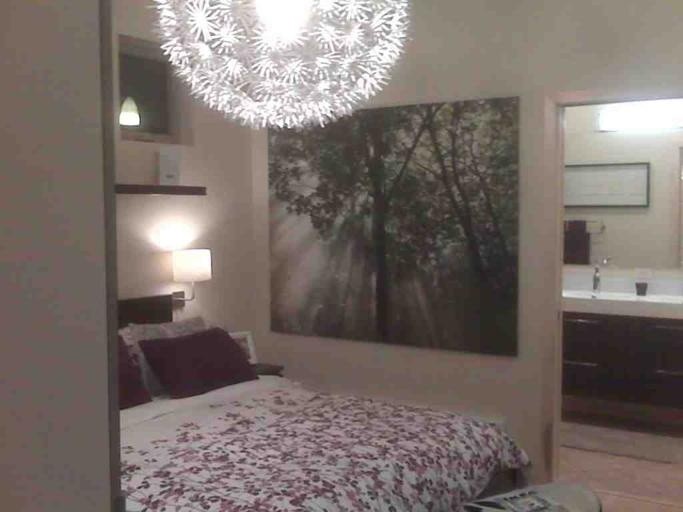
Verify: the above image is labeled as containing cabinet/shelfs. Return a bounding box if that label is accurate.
[563,311,682,409]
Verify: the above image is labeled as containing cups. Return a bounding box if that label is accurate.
[635,282,648,296]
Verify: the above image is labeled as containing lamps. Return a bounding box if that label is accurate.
[170,248,213,309]
[150,0,409,135]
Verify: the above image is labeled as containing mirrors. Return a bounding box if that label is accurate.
[564,130,683,270]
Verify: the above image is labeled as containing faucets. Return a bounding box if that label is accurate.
[593,264,601,298]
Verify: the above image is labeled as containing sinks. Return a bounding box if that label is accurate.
[563,290,637,301]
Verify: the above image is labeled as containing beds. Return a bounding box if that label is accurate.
[119,294,527,512]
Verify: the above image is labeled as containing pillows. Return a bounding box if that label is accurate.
[119,315,258,410]
